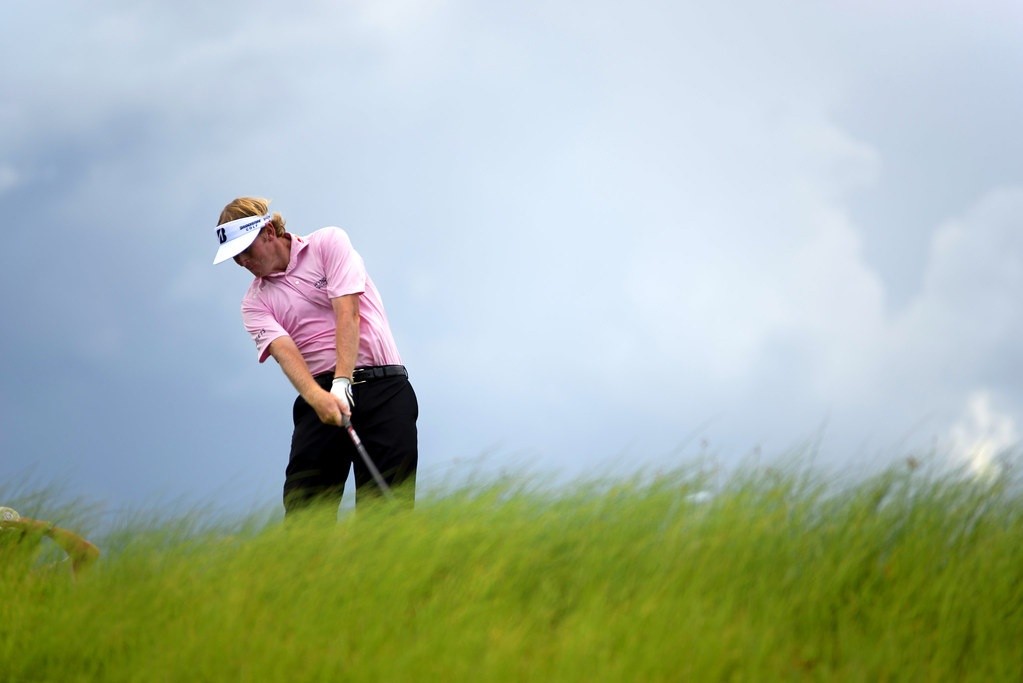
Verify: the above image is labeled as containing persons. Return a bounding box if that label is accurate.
[212,197,419,531]
[0,506,101,589]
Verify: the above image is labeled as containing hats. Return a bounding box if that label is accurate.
[213,212,272,265]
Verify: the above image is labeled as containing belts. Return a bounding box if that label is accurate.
[351,365,407,384]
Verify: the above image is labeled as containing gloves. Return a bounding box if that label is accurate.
[330,379,354,414]
